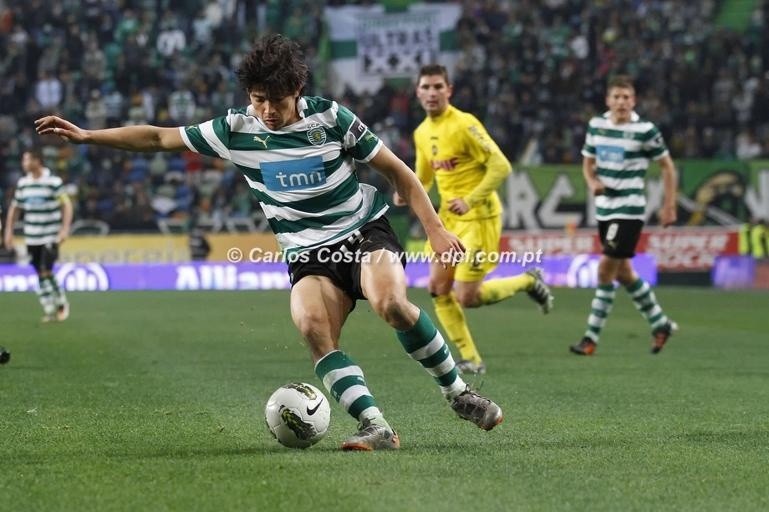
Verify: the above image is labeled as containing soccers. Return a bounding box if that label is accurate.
[263,382,331,448]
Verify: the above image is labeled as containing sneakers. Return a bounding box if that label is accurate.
[456,361,489,376]
[652,319,678,354]
[526,267,554,315]
[569,337,596,356]
[450,390,504,432]
[341,417,400,451]
[39,295,70,322]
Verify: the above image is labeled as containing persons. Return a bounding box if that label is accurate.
[0,0,768,264]
[33,33,504,449]
[567,74,678,356]
[393,63,554,375]
[2,147,73,322]
